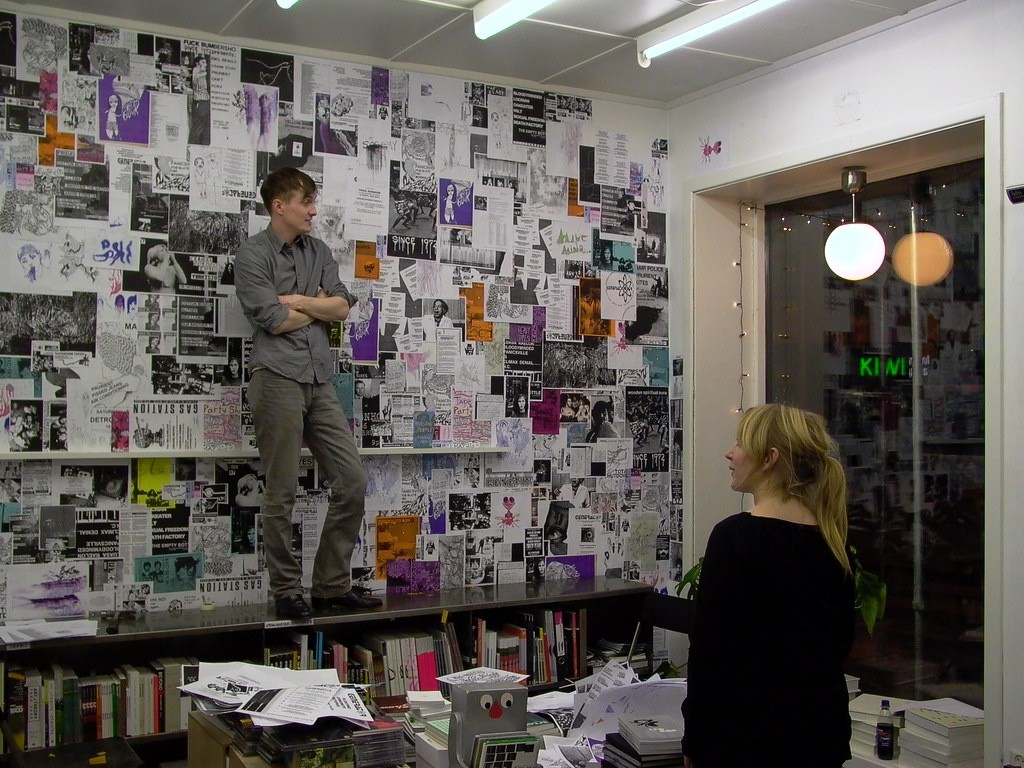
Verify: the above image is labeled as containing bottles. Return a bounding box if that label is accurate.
[875,700,894,761]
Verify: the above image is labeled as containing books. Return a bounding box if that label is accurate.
[0,602,685,768]
[844,674,985,768]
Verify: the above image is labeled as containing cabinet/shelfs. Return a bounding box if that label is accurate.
[0,577,654,754]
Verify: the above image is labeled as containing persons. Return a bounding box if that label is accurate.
[511,393,528,419]
[534,393,671,580]
[423,300,453,340]
[143,244,187,295]
[233,167,383,620]
[681,403,855,768]
[220,357,242,386]
[238,525,256,554]
[221,261,236,285]
[391,177,519,245]
[598,246,616,271]
[235,473,265,507]
[355,376,386,415]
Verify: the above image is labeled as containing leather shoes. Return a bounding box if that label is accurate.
[311,591,383,612]
[275,594,314,620]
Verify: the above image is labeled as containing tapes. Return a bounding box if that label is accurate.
[200,602,214,611]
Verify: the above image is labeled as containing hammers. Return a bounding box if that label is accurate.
[100,608,136,634]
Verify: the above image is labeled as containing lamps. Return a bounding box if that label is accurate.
[824,166,885,281]
[473,0,557,40]
[636,0,785,68]
[891,172,952,288]
